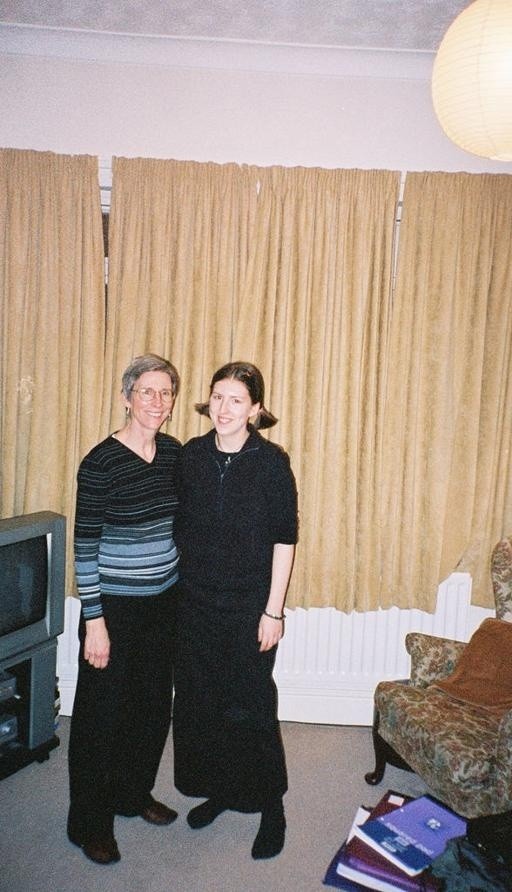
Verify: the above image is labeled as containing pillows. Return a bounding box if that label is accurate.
[425,617,512,714]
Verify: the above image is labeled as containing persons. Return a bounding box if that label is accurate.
[72,353,177,868]
[173,364,299,860]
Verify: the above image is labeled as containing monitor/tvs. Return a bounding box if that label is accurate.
[0,509,69,662]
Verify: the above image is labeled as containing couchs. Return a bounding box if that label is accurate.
[364,533,512,852]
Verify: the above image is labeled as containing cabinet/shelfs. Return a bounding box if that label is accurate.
[0,637,60,781]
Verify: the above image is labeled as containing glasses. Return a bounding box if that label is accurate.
[127,386,177,402]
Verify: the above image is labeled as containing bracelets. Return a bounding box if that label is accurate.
[262,610,286,620]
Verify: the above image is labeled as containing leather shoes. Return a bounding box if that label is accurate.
[66,829,121,864]
[116,795,178,826]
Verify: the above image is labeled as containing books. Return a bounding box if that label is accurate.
[321,789,468,891]
